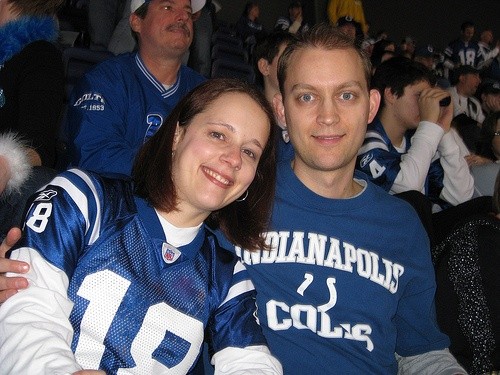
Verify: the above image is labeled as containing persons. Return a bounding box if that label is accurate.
[1,0,500,233]
[0,20,467,375]
[0,76,279,375]
[66,0,208,178]
